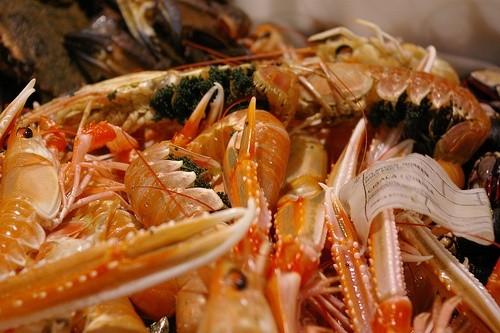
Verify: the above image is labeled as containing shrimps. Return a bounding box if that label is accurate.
[0,18,500,333]
